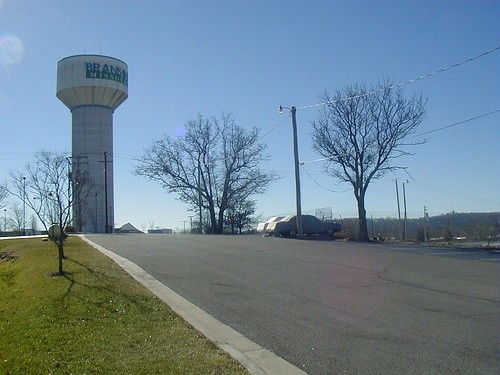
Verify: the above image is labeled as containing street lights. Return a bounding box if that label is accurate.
[97,151,113,233]
[4,209,6,231]
[278,105,303,239]
[22,176,26,236]
[402,179,408,239]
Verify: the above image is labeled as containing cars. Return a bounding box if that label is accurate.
[256,216,286,236]
[266,214,342,238]
